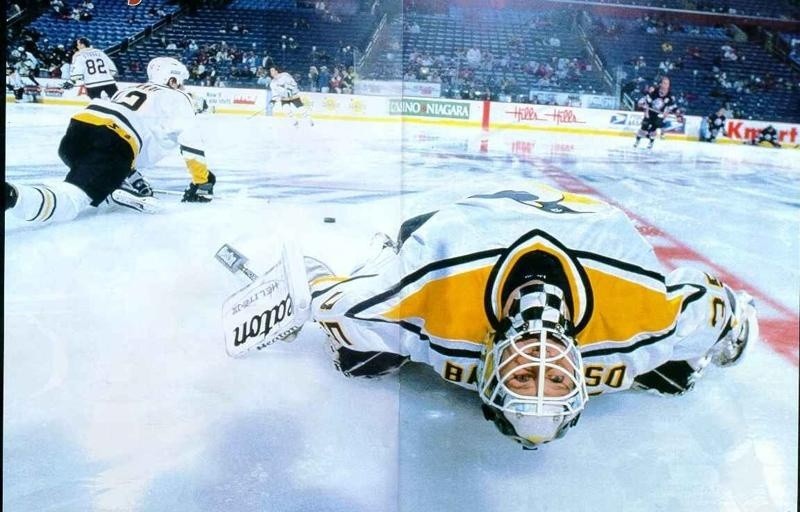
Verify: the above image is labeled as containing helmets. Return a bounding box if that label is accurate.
[476,324,591,453]
[146,57,190,86]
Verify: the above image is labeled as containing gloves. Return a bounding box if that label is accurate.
[181,170,215,202]
[62,78,76,90]
[129,171,154,196]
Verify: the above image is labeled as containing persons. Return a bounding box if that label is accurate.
[4,56,217,224]
[302,177,759,451]
[4,0,799,150]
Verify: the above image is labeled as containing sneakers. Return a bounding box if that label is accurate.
[112,187,162,213]
[634,141,654,148]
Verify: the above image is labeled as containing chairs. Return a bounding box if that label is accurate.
[27,1,800,130]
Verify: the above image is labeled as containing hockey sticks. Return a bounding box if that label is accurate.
[153,186,249,201]
[47,82,84,89]
[244,103,271,120]
